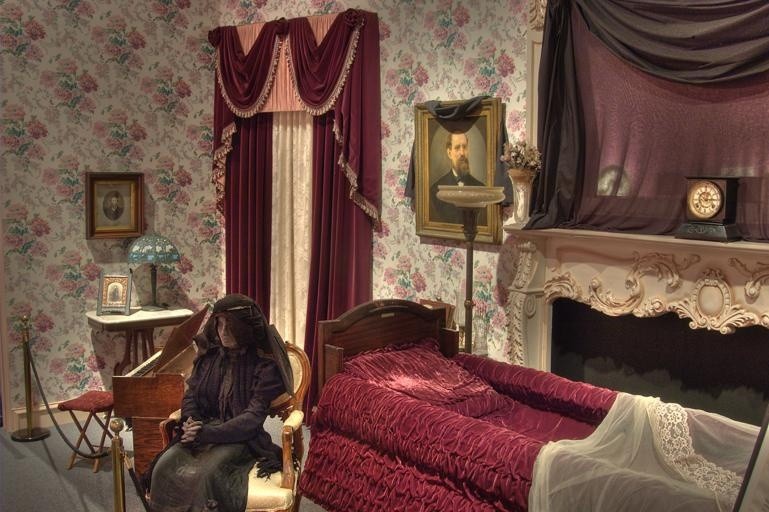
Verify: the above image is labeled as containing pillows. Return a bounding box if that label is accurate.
[343,337,507,418]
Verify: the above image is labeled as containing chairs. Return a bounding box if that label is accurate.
[159,341,311,512]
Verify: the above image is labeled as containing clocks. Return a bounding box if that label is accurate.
[673,176,741,242]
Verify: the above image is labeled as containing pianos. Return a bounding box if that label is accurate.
[111,304,210,483]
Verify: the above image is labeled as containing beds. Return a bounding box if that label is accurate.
[297,298,768,511]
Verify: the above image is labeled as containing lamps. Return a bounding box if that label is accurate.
[128,233,181,311]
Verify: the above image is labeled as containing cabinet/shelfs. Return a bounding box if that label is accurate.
[414,98,505,248]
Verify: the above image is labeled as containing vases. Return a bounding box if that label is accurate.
[508,169,539,225]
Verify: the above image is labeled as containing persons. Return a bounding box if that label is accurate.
[104,191,123,220]
[149,293,293,512]
[430,129,487,226]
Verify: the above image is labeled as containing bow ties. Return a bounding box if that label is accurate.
[455,174,467,183]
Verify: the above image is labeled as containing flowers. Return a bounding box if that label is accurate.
[499,139,542,170]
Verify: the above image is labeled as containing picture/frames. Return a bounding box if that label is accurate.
[83,171,145,241]
[95,271,133,315]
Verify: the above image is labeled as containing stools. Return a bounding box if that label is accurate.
[57,390,122,473]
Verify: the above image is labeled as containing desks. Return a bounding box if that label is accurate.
[85,306,193,376]
